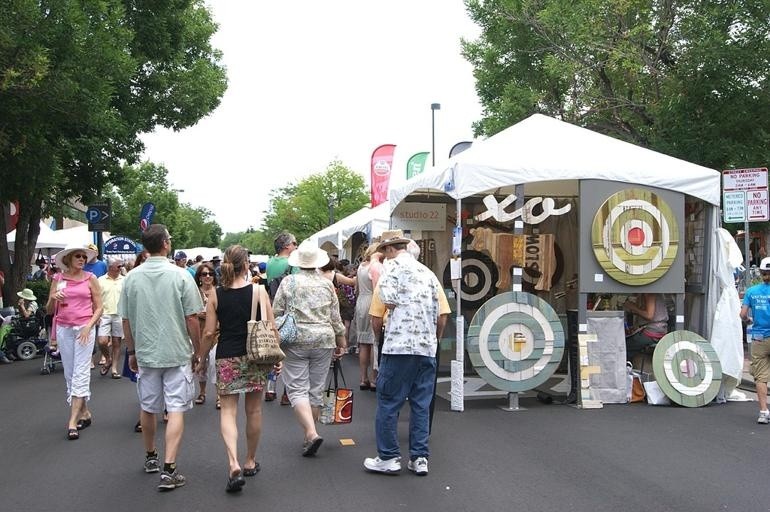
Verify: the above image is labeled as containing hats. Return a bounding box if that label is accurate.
[377,229,410,253]
[17,288,37,300]
[759,257,770,269]
[288,240,329,268]
[210,256,222,261]
[173,251,186,260]
[55,242,97,270]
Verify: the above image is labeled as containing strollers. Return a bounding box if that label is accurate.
[39,314,61,374]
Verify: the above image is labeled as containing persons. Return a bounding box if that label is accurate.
[46,241,103,440]
[195,245,280,491]
[363,229,439,473]
[750,238,762,263]
[119,225,204,489]
[0,249,452,436]
[738,255,770,424]
[623,293,670,368]
[266,233,300,302]
[271,242,347,455]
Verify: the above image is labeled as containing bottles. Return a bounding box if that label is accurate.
[266,370,277,395]
[745,321,754,344]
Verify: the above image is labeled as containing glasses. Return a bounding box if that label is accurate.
[200,271,214,277]
[74,254,88,259]
[117,264,125,268]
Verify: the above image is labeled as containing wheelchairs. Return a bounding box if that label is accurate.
[1,307,46,361]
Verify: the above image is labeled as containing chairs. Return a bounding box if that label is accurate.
[639,314,676,375]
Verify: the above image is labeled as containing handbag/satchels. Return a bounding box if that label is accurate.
[273,314,297,343]
[270,270,292,299]
[627,371,671,404]
[247,320,286,365]
[36,257,46,265]
[318,388,353,424]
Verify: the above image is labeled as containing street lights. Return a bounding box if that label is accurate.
[174,187,186,197]
[325,193,336,225]
[429,102,442,168]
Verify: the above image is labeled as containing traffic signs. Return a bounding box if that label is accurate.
[720,167,769,191]
[746,189,770,222]
[85,196,112,232]
[722,190,747,224]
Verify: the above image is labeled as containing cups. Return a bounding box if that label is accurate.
[60,293,69,308]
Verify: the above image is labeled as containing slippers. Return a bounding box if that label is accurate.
[303,435,323,456]
[99,359,122,379]
[68,418,91,438]
[226,475,245,492]
[244,462,260,475]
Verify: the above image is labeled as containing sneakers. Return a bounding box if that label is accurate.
[216,397,221,409]
[360,377,377,391]
[158,472,185,488]
[281,394,290,404]
[757,409,770,423]
[265,391,277,400]
[363,455,401,473]
[407,457,428,473]
[195,393,205,403]
[144,455,162,472]
